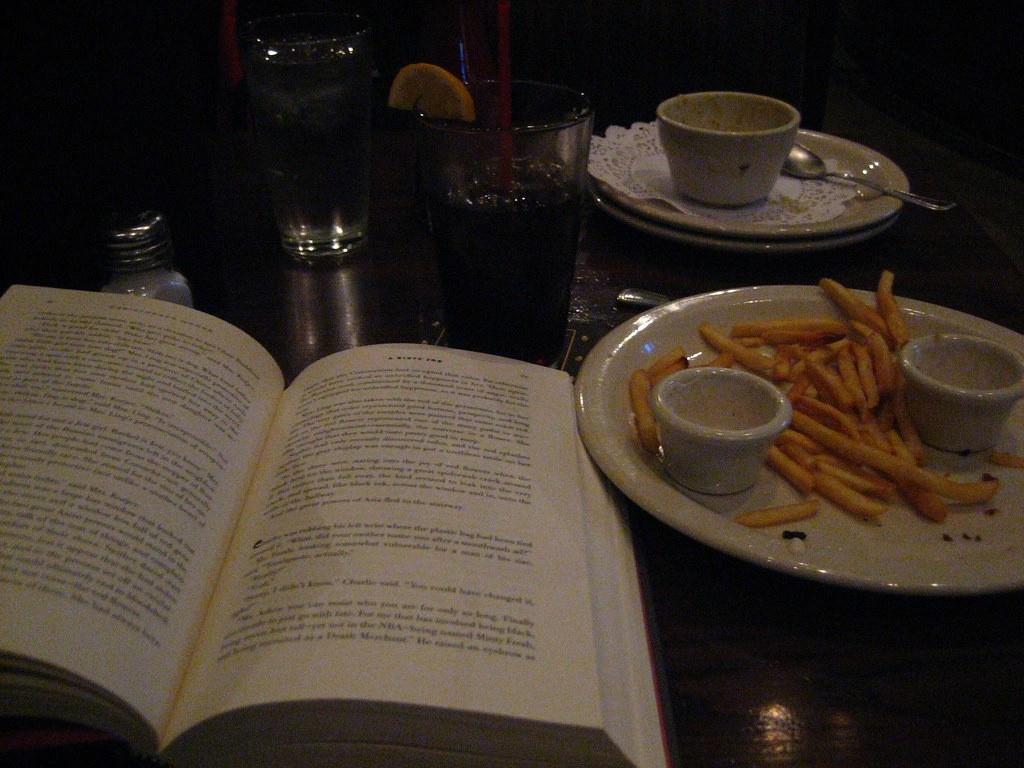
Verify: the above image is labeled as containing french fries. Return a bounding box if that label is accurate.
[628,268,1024,529]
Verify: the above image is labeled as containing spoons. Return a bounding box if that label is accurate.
[781,143,956,210]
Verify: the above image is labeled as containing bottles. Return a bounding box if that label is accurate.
[86,209,193,310]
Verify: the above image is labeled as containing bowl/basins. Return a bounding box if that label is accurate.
[648,367,791,495]
[897,332,1024,453]
[656,92,799,206]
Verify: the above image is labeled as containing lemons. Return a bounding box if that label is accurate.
[388,62,476,121]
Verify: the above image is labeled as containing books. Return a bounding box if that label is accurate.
[0,283,671,768]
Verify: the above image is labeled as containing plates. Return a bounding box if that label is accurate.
[588,128,911,249]
[572,284,1024,596]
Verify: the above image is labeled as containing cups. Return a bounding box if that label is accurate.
[417,80,596,364]
[241,11,375,257]
